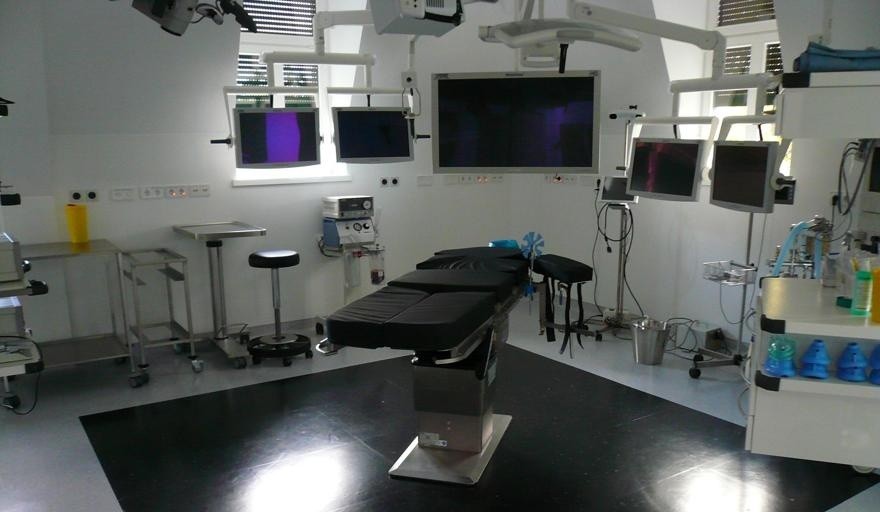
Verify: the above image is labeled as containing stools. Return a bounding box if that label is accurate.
[531,255,602,354]
[246,250,313,367]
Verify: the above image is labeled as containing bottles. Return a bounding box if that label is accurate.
[868,263,880,324]
[849,260,871,316]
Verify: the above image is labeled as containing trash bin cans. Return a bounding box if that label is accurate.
[632,319,668,365]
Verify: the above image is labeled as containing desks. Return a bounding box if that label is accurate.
[172,221,266,368]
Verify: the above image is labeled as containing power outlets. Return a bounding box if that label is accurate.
[544,175,577,185]
[593,175,605,188]
[140,185,210,199]
[460,173,504,185]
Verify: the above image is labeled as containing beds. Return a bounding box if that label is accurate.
[316,245,533,484]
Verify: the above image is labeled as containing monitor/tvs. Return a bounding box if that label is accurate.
[626,137,704,202]
[429,70,603,176]
[597,175,639,206]
[332,106,414,165]
[710,140,778,214]
[232,107,321,168]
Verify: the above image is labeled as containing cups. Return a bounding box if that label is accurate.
[64,202,90,245]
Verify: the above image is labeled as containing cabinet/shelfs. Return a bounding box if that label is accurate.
[0,97,48,410]
[744,68,879,474]
